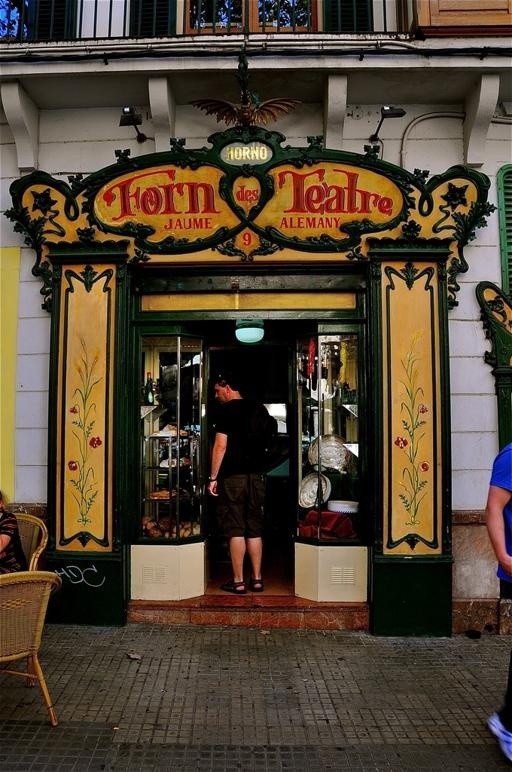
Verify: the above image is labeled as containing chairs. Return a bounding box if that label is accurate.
[0,571,65,727]
[13,511,50,571]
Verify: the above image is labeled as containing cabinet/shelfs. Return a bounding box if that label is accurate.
[286,321,368,604]
[124,324,210,602]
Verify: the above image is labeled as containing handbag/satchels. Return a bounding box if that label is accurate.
[239,406,279,474]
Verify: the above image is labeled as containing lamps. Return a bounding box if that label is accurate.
[368,104,407,148]
[116,103,149,145]
[235,318,266,344]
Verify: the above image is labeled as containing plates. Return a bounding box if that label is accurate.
[307,435,350,473]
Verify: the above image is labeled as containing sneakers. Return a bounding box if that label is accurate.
[486,711,512,762]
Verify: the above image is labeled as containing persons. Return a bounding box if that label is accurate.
[0,489,29,575]
[485,442,512,762]
[207,375,273,594]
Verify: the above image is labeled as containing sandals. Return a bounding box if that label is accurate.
[221,577,249,593]
[247,577,264,592]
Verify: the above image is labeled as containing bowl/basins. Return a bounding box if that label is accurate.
[158,473,168,483]
[300,473,331,508]
[328,501,359,514]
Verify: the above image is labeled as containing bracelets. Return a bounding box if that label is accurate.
[210,479,217,482]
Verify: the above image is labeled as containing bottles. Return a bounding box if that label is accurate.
[144,372,157,406]
